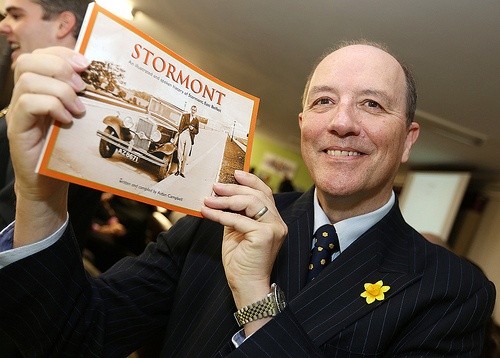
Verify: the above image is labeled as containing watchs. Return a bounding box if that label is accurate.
[234,282,287,327]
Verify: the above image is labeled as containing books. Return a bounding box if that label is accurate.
[32,1,261,223]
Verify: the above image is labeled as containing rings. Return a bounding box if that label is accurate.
[254,206,268,220]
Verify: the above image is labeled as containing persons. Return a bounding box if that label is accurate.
[174,105,199,179]
[0,0,98,232]
[0,44,497,358]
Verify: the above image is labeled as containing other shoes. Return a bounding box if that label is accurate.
[175,171,179,176]
[180,173,185,178]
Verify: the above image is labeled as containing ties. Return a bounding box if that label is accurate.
[306,224,341,284]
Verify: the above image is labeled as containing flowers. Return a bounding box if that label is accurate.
[360,280,391,304]
